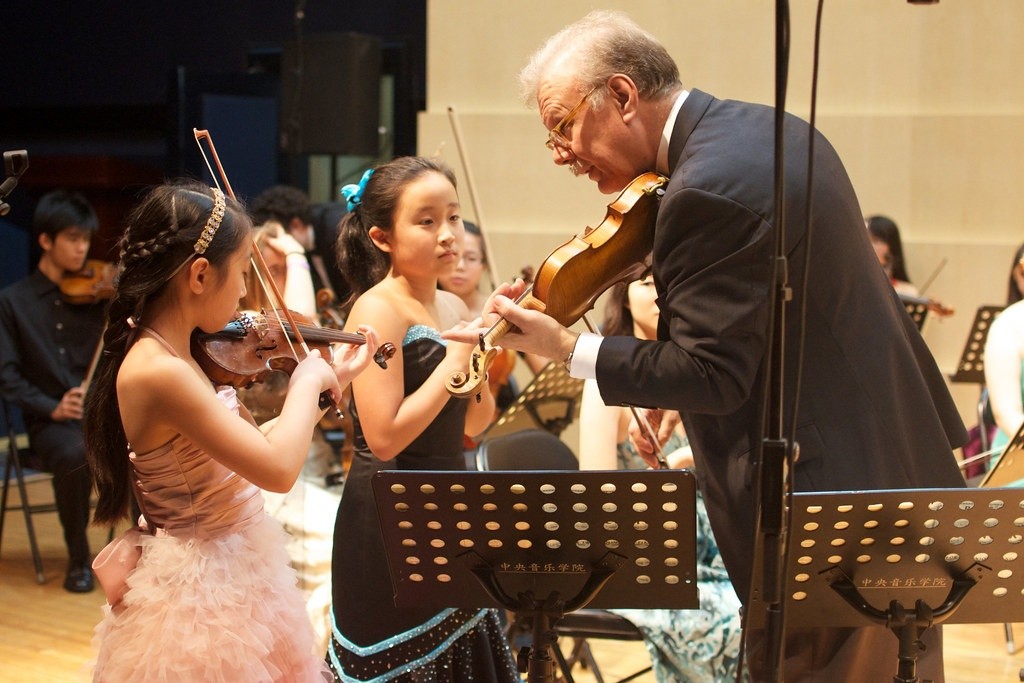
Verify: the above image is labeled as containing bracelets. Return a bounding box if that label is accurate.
[285,252,305,256]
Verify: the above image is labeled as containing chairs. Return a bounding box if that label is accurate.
[476,429,646,683]
[0,392,118,584]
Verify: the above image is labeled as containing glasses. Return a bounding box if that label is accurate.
[456,252,485,267]
[546,85,606,152]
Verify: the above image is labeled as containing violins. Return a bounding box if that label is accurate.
[926,299,954,317]
[462,348,516,451]
[190,307,397,389]
[59,259,118,304]
[445,171,671,404]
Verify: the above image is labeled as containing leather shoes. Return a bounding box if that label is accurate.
[64,555,94,592]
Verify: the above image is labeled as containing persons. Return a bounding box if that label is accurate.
[578,275,749,683]
[239,221,316,321]
[0,193,140,593]
[440,15,968,683]
[982,239,1024,487]
[80,182,379,683]
[250,186,358,312]
[436,221,518,409]
[324,158,519,683]
[863,215,920,309]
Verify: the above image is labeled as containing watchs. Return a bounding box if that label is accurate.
[563,333,581,372]
